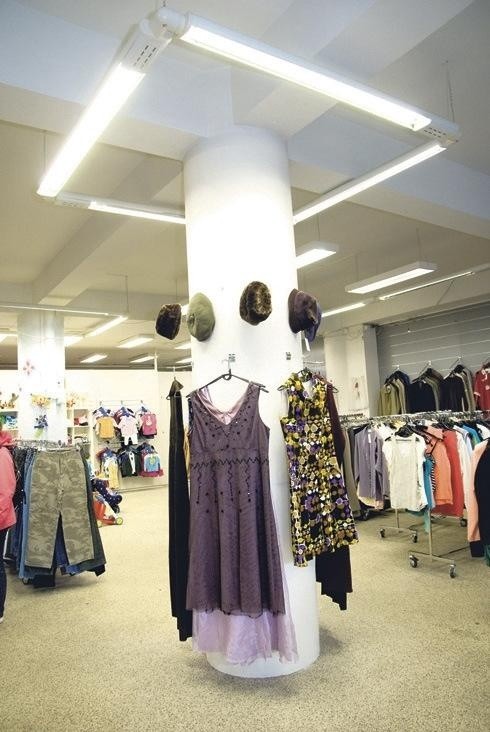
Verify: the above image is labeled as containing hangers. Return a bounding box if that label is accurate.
[419,362,434,376]
[187,356,266,394]
[337,414,364,422]
[482,355,489,367]
[367,414,409,424]
[277,361,338,394]
[399,410,490,423]
[17,439,80,451]
[448,358,461,371]
[387,365,398,383]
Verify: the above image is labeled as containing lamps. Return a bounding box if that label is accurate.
[292,141,448,226]
[35,22,172,201]
[175,276,188,316]
[51,193,187,226]
[173,338,191,351]
[344,263,439,296]
[175,354,190,369]
[152,6,465,147]
[295,244,341,270]
[113,333,156,349]
[71,352,109,363]
[130,351,156,363]
[1,329,84,342]
[85,276,130,338]
[375,270,474,300]
[320,299,369,317]
[0,300,121,319]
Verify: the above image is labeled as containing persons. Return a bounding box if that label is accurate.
[0,422,18,622]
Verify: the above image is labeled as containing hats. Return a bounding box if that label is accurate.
[287,288,318,334]
[239,281,272,326]
[185,293,216,342]
[155,303,182,340]
[304,304,322,343]
